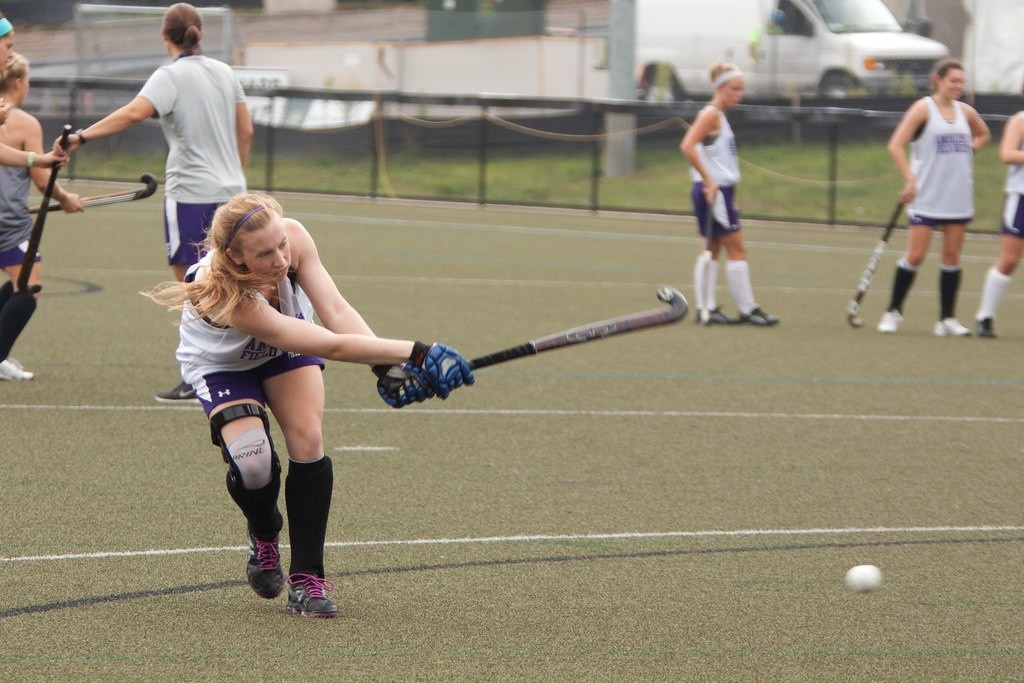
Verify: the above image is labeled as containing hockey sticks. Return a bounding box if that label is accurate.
[845,201,904,327]
[700,204,712,325]
[16,125,73,296]
[27,172,158,215]
[388,286,689,394]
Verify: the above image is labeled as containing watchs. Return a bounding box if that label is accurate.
[75,127,87,144]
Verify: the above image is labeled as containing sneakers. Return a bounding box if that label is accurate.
[246,523,284,599]
[933,317,971,337]
[155,380,200,403]
[287,573,338,619]
[0,357,34,382]
[741,307,779,326]
[976,317,998,338]
[699,308,742,325]
[878,309,904,333]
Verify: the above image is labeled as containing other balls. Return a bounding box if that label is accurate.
[846,564,882,592]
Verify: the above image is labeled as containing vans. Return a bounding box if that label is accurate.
[632,0,954,144]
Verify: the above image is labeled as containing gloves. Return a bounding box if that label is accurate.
[409,341,474,399]
[371,363,435,408]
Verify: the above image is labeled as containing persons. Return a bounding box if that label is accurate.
[138,191,474,619]
[975,89,1024,338]
[680,61,783,327]
[52,1,254,404]
[877,58,992,340]
[0,9,86,385]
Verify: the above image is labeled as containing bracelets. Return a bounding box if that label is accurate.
[27,151,35,168]
